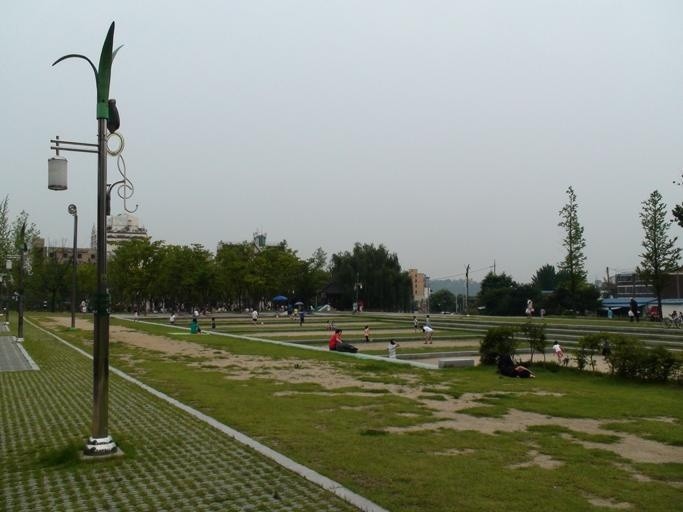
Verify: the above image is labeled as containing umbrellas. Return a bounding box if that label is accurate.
[273,295,288,301]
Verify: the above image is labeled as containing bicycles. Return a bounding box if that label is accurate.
[660,313,682,329]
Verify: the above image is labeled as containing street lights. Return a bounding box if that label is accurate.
[353,271,363,304]
[5,247,25,342]
[65,202,77,328]
[42,20,132,463]
[423,276,431,314]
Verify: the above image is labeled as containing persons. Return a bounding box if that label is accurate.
[628,297,641,322]
[353,300,364,312]
[252,309,258,325]
[413,315,435,344]
[329,329,358,353]
[325,320,336,330]
[170,312,176,325]
[540,308,546,319]
[189,309,202,334]
[388,339,400,359]
[79,298,88,317]
[552,340,564,363]
[608,307,613,318]
[500,366,535,379]
[364,326,369,342]
[287,304,305,328]
[526,297,534,318]
